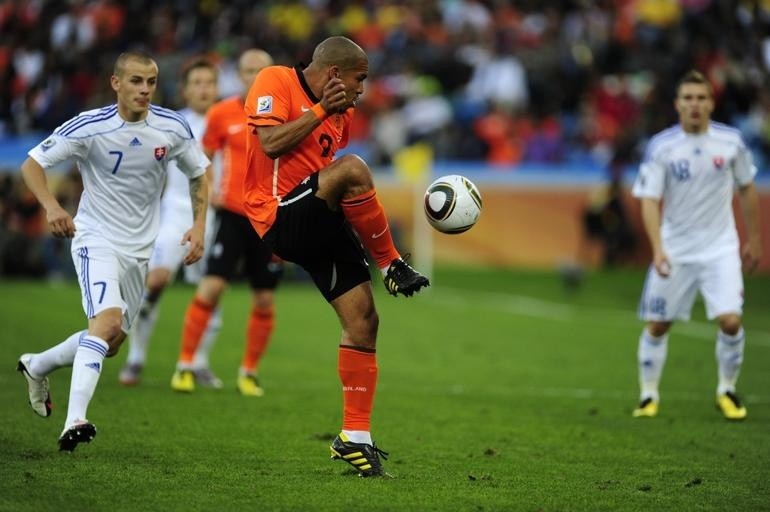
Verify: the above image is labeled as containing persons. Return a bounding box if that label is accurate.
[240,36,431,477]
[628,70,760,422]
[170,49,292,398]
[0,1,769,251]
[117,61,233,392]
[20,49,212,455]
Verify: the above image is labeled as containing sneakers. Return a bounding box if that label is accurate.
[714,391,748,422]
[56,421,97,452]
[14,352,53,418]
[116,361,266,399]
[631,399,660,418]
[379,256,430,298]
[328,430,390,477]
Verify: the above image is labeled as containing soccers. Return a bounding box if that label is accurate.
[424,175,484,235]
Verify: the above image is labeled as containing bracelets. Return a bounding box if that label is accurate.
[310,102,330,122]
